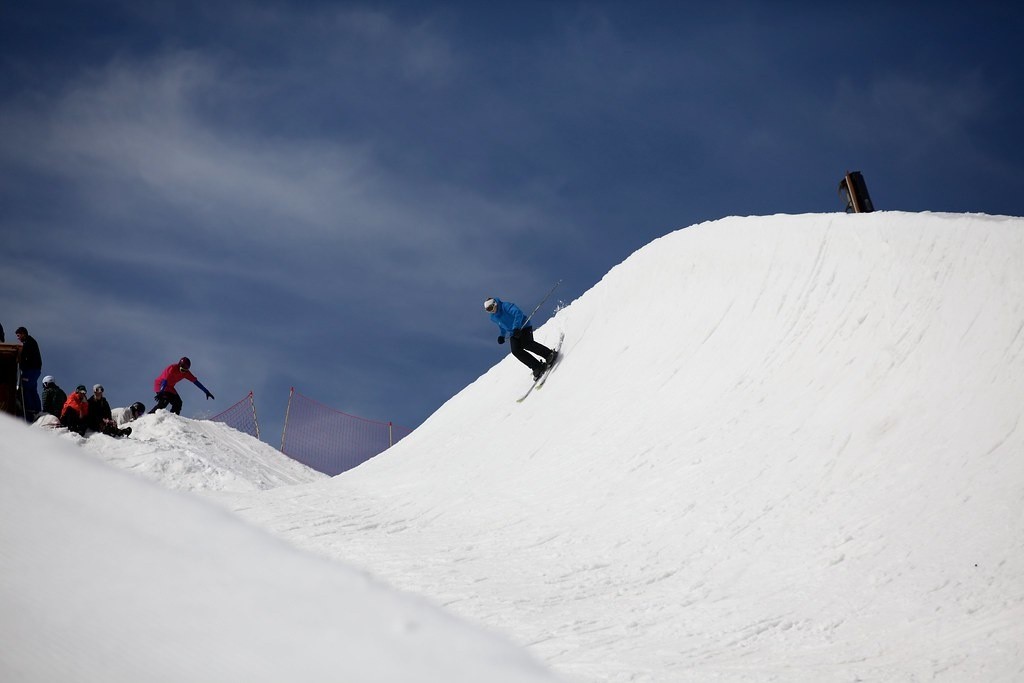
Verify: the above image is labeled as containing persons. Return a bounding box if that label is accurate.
[147,357,214,416]
[111,402,145,426]
[484,298,558,381]
[87,384,132,437]
[41,376,67,418]
[14,327,42,422]
[58,384,88,437]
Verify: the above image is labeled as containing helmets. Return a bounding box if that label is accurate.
[93,384,104,393]
[76,385,87,394]
[177,357,191,368]
[130,402,145,415]
[42,375,56,383]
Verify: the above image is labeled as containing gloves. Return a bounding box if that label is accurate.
[205,392,214,400]
[498,336,505,344]
[153,392,163,402]
[513,328,522,338]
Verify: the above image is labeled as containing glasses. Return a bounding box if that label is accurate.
[483,300,498,311]
[179,366,186,372]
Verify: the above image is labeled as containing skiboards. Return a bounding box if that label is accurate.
[516,332,565,402]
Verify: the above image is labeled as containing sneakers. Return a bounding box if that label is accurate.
[533,359,547,381]
[546,349,559,370]
